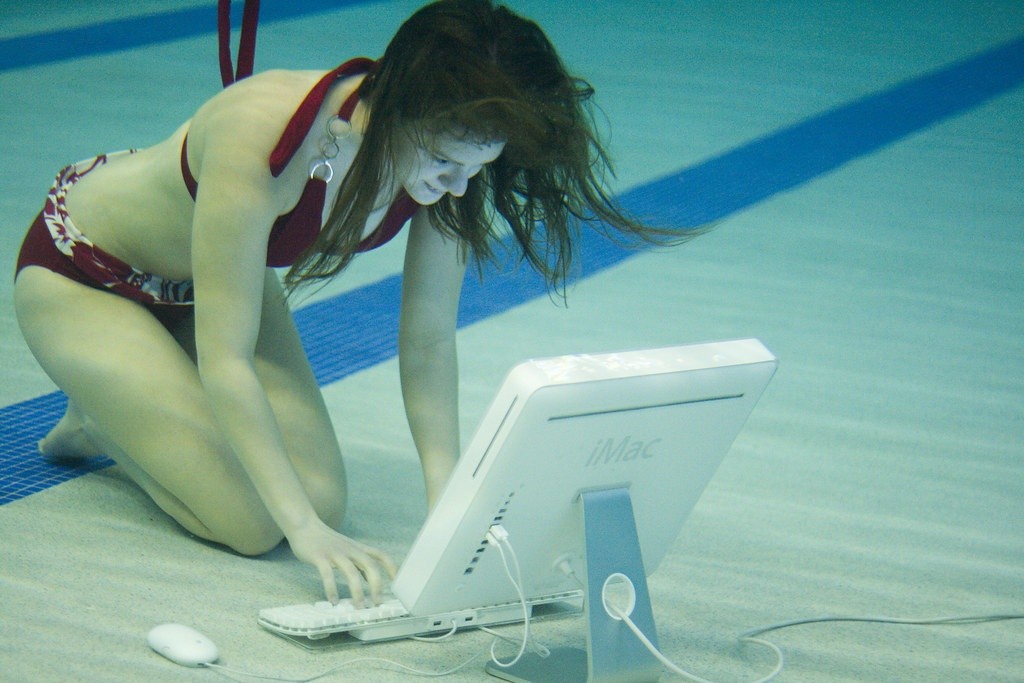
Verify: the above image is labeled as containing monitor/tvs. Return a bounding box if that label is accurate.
[392,337,781,683]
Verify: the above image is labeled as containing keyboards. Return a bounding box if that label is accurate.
[255,590,584,644]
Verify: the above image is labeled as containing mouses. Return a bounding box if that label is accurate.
[147,623,219,668]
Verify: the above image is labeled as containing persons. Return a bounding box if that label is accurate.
[11,0,699,610]
